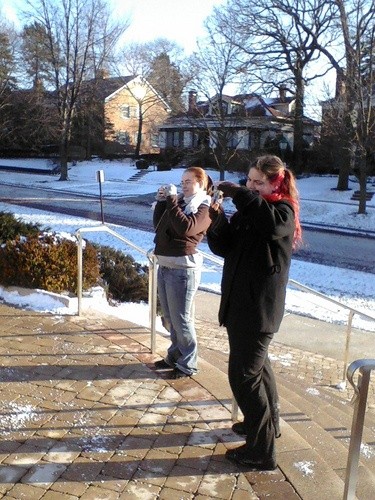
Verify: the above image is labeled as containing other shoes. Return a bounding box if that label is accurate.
[225,448,277,470]
[232,422,248,435]
[155,359,170,368]
[167,369,190,380]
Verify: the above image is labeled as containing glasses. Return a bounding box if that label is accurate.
[180,180,194,185]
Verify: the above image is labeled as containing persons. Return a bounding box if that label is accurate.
[153,167,214,378]
[206,155,302,470]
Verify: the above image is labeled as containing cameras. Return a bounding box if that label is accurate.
[211,190,224,205]
[163,185,170,194]
